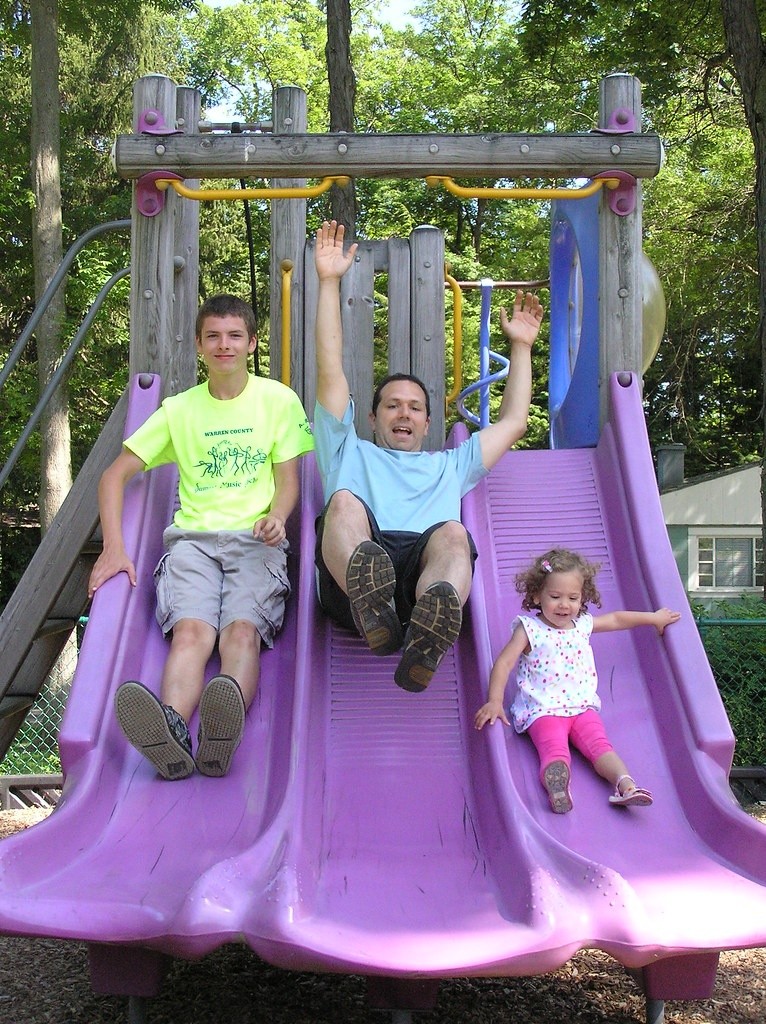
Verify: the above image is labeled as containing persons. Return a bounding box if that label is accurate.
[473,550,682,815]
[314,218,544,692]
[87,295,315,781]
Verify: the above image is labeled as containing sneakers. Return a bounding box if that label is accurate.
[114,674,246,781]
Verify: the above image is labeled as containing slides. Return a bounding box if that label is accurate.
[1,367,766,1002]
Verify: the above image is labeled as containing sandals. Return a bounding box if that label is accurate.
[542,759,574,813]
[608,776,654,806]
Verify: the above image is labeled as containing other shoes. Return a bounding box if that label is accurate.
[394,581,462,693]
[345,541,405,656]
